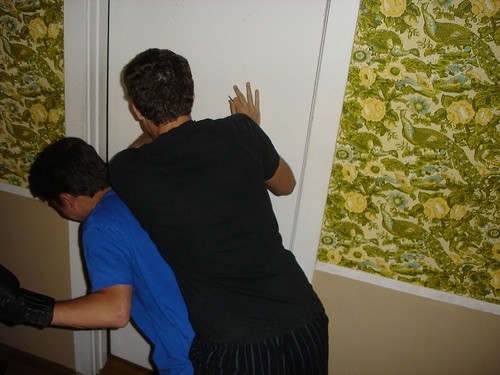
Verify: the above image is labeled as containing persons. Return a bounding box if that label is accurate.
[106,47,329,375]
[0,137,197,375]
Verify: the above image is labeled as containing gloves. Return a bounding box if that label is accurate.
[0,264,56,329]
